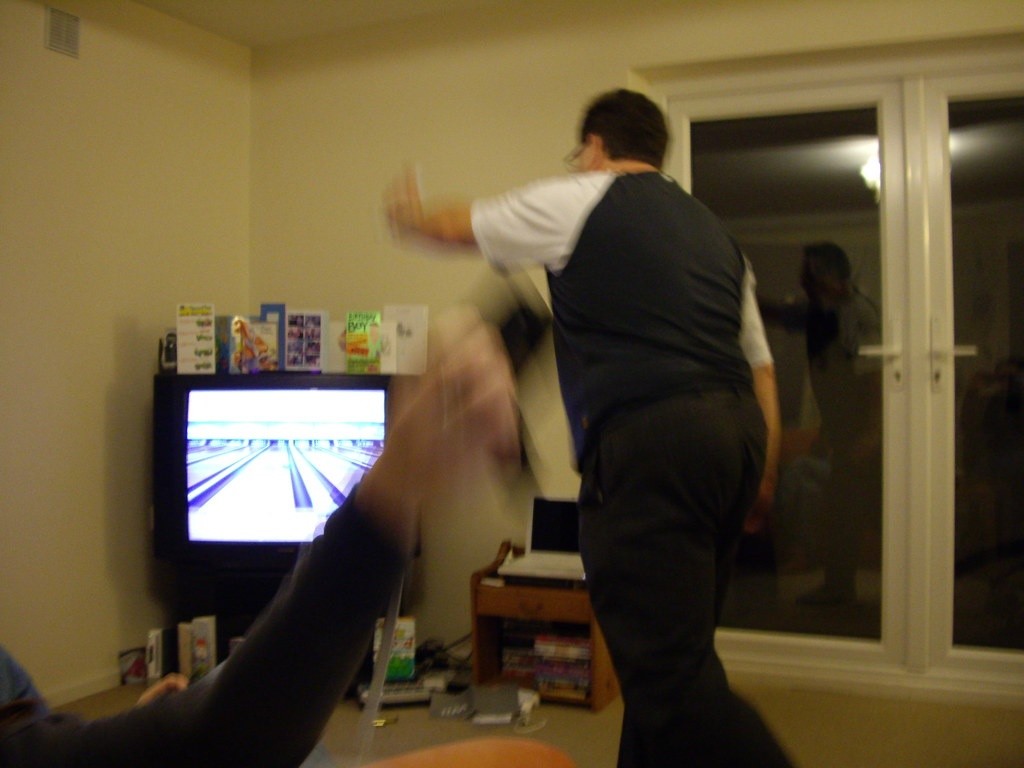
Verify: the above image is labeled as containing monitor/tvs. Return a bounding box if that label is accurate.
[151,371,425,575]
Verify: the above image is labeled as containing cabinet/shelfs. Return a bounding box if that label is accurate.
[468,536,623,715]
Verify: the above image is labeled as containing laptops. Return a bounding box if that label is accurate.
[497,492,587,583]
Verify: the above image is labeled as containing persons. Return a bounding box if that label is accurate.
[754,239,882,608]
[0,326,523,768]
[381,89,809,767]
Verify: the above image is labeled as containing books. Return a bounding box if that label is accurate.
[374,616,416,680]
[141,616,215,683]
[346,304,429,374]
[428,631,592,723]
